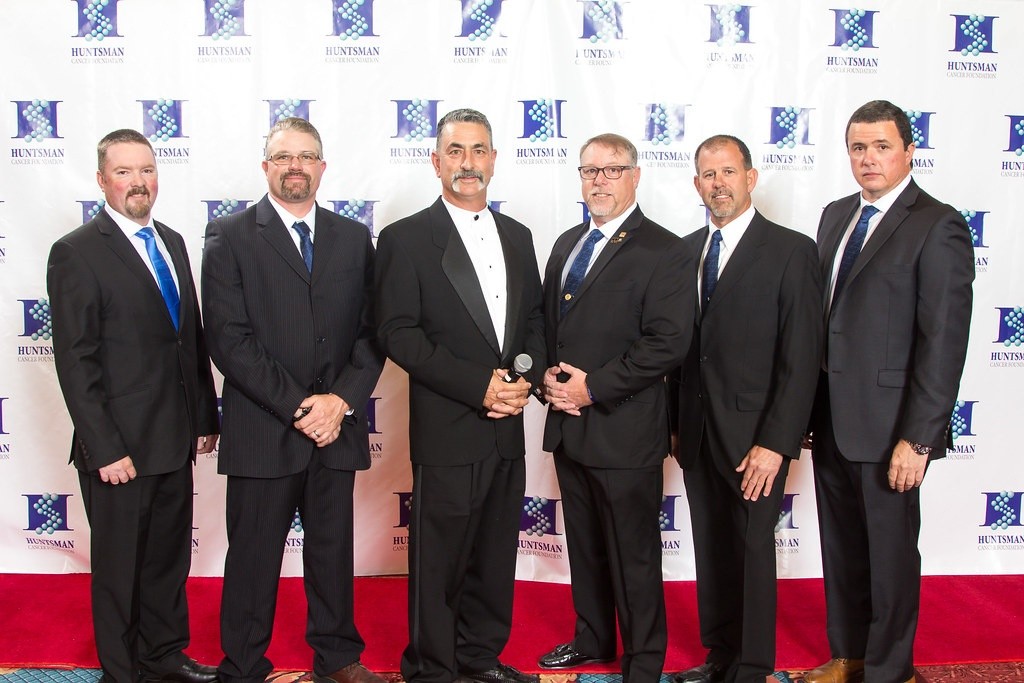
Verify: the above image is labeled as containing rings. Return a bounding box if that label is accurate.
[314,430,321,438]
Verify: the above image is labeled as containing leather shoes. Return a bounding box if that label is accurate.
[673,662,723,683]
[165,659,219,683]
[538,643,616,669]
[312,661,386,683]
[465,662,540,683]
[805,657,864,683]
[906,676,916,682]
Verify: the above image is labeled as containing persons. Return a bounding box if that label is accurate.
[799,100,975,683]
[201,117,389,682]
[667,134,822,683]
[530,133,695,683]
[45,127,225,683]
[373,108,543,683]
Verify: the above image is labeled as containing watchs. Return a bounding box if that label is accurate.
[905,440,933,456]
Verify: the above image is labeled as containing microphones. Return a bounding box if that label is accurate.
[478,353,533,420]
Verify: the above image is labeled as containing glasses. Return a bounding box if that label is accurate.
[578,166,631,180]
[268,153,319,165]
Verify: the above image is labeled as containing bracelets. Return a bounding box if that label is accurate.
[296,408,312,422]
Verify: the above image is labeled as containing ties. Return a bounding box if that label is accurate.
[701,230,723,320]
[561,229,605,318]
[832,206,880,304]
[291,221,314,273]
[135,227,180,332]
